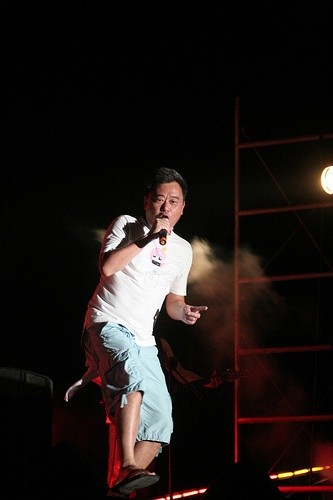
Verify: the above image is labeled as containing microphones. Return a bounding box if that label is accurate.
[159,215,167,245]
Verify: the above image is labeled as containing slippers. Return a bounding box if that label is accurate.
[111,469,159,493]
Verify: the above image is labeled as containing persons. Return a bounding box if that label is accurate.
[64,334,222,498]
[81,169,208,500]
